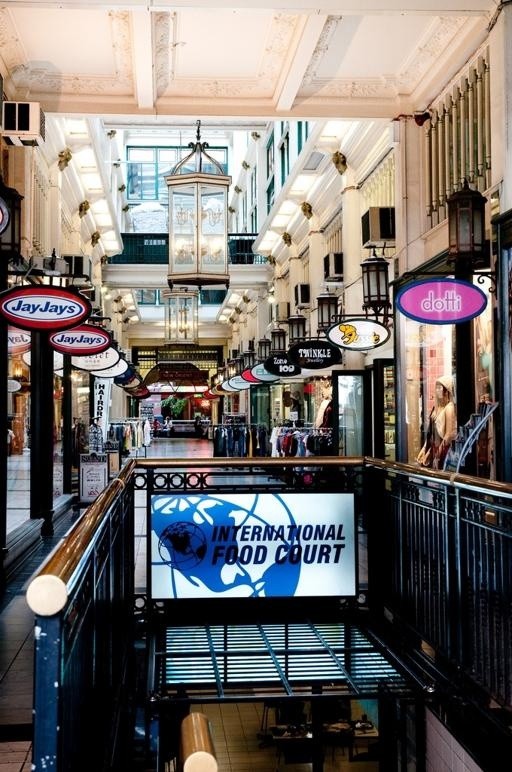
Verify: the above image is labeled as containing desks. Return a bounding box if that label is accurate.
[261,699,379,772]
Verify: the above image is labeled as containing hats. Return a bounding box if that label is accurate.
[435,374,454,396]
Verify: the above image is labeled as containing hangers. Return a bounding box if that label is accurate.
[271,426,335,441]
[216,422,265,430]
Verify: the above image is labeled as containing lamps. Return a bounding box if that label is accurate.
[164,119,233,282]
[164,286,199,348]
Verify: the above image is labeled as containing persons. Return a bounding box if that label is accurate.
[422,374,455,470]
[415,440,427,464]
[164,416,172,437]
[290,388,332,433]
[152,419,160,440]
[194,416,204,438]
[90,414,104,453]
[6,423,59,457]
